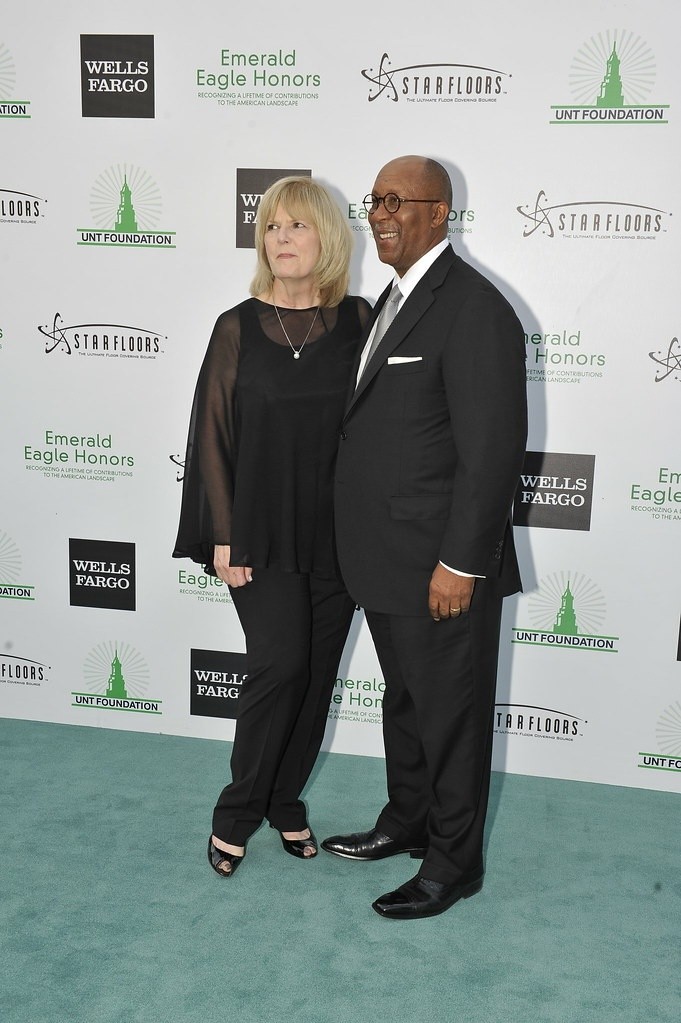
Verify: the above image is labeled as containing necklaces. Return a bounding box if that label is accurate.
[268,289,320,358]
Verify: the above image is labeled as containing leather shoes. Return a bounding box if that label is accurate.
[321,827,427,861]
[372,874,483,920]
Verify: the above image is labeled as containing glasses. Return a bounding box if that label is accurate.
[362,193,441,214]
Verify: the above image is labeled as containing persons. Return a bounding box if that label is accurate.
[169,175,373,877]
[317,155,530,922]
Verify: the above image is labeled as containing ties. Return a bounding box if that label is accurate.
[360,284,403,377]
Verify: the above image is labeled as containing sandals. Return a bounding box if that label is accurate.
[207,834,246,877]
[269,822,318,859]
